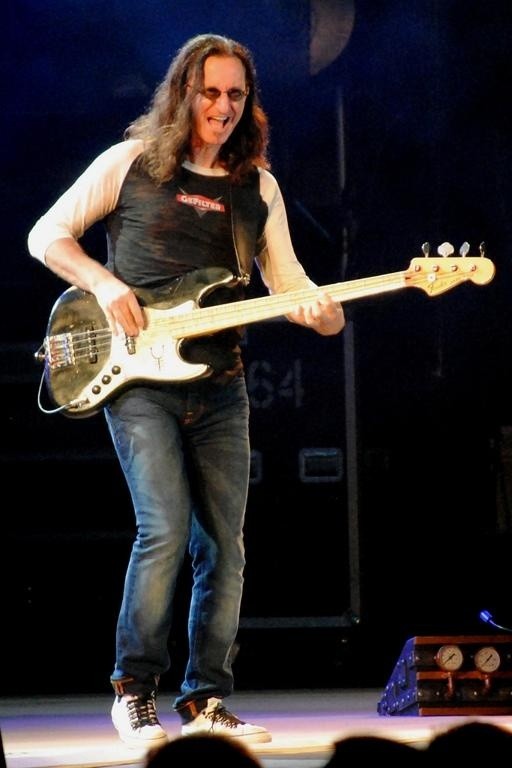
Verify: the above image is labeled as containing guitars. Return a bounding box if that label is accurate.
[44,241,495,418]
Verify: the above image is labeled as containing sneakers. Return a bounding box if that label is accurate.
[110,690,167,748]
[181,699,272,746]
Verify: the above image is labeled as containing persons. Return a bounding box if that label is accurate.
[145,731,263,768]
[27,32,346,747]
[322,721,512,768]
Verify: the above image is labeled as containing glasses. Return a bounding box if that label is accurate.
[185,80,250,104]
